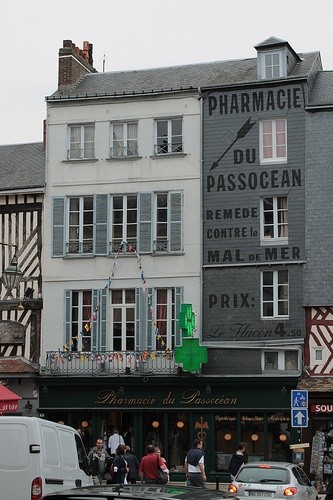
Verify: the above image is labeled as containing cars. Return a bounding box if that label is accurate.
[228,462,319,500]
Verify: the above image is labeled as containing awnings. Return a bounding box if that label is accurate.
[0,383,22,416]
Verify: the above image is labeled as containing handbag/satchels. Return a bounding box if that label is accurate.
[155,456,169,486]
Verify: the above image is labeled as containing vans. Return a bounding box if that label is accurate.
[0,415,100,500]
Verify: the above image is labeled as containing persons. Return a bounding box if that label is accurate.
[228,442,246,483]
[88,424,169,485]
[185,439,206,488]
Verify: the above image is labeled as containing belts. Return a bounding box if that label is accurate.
[188,472,202,475]
